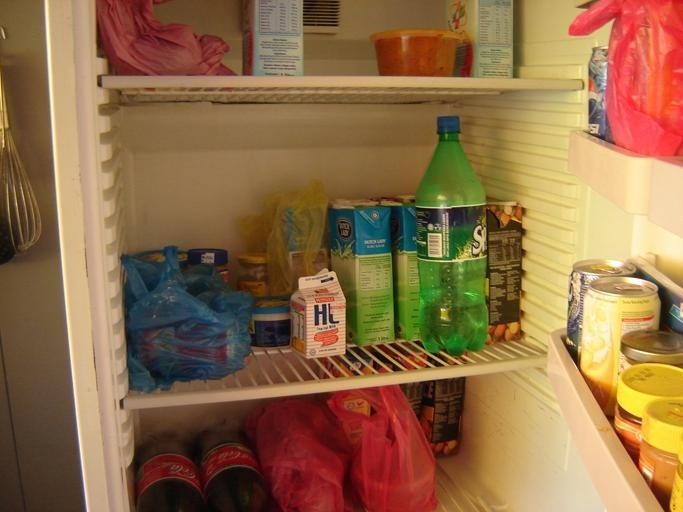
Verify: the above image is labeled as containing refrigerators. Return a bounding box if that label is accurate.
[40,1,682,512]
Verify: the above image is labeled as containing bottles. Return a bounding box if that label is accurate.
[588,47,608,140]
[249,296,291,346]
[615,363,683,466]
[580,276,661,418]
[185,248,229,287]
[416,116,489,355]
[618,329,683,376]
[670,442,683,504]
[136,428,204,512]
[639,399,682,504]
[566,259,637,363]
[237,253,269,298]
[198,423,269,512]
